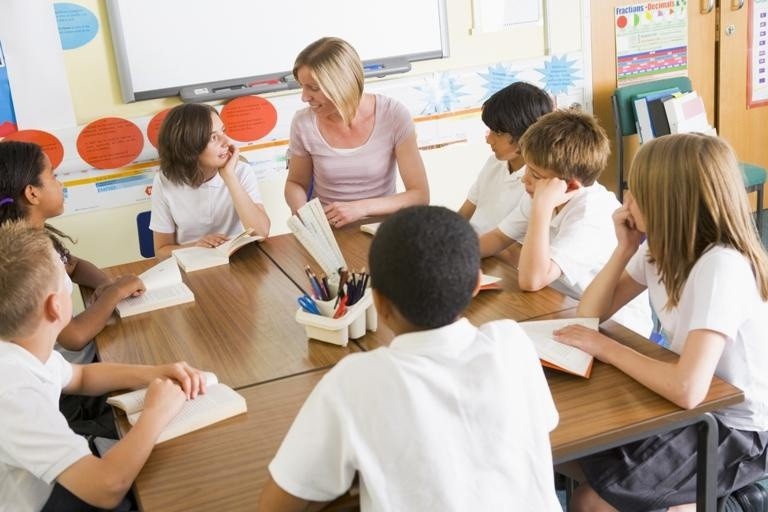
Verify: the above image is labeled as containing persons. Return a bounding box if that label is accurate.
[552,133,767,512]
[456,82,555,267]
[259,204,563,512]
[0,141,146,415]
[1,218,206,512]
[148,103,270,259]
[284,36,430,229]
[479,107,655,339]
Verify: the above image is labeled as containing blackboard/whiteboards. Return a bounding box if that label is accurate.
[105,0,450,106]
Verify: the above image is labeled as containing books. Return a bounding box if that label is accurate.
[171,223,266,273]
[478,255,504,296]
[105,365,248,447]
[360,221,383,235]
[512,316,600,378]
[116,256,194,319]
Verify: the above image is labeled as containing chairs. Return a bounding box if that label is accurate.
[137,210,155,258]
[611,76,767,237]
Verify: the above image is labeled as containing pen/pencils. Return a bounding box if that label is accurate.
[306,263,326,299]
[338,268,369,306]
[303,264,322,300]
[307,272,322,299]
[318,275,328,299]
[321,271,330,299]
[311,271,326,300]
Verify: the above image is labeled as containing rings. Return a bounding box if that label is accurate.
[332,217,339,225]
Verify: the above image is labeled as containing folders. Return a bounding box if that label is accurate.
[649,92,689,136]
[633,90,679,144]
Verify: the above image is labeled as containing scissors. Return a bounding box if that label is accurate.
[331,296,348,319]
[297,295,321,316]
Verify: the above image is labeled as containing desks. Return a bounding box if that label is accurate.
[77,240,365,417]
[116,306,746,512]
[257,209,580,358]
